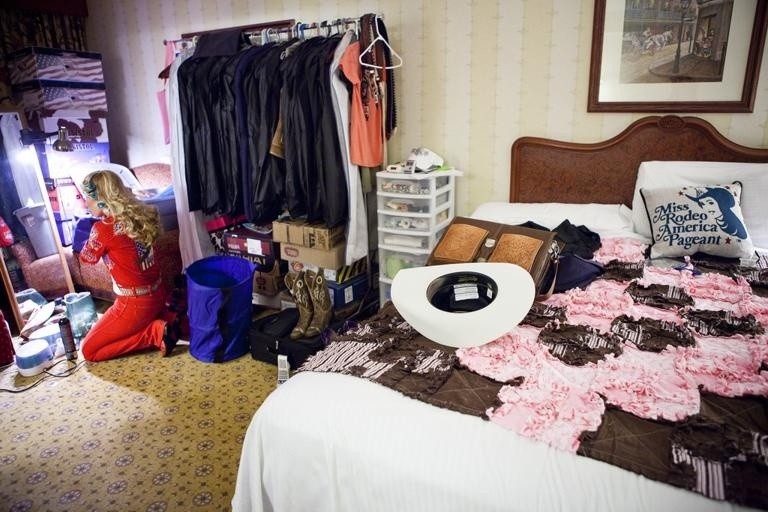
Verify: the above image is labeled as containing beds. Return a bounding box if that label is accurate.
[228,115,768,512]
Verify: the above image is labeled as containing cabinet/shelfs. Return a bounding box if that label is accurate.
[376,170,455,310]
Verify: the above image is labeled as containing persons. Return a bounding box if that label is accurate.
[79,170,183,362]
[685,187,747,239]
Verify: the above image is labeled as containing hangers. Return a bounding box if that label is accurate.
[166,15,402,69]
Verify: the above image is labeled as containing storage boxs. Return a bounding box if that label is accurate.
[203,214,369,316]
[6,49,111,247]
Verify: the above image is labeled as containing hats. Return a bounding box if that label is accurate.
[391,258,536,349]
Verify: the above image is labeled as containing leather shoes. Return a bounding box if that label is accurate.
[159,314,181,357]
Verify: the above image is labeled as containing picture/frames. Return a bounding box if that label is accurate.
[587,0,768,114]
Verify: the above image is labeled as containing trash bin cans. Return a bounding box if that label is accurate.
[186,255,257,363]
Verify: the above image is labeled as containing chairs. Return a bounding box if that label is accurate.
[10,242,70,299]
[62,162,183,302]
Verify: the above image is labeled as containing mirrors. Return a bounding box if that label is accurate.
[0,112,74,343]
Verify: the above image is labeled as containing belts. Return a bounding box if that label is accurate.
[112,272,163,296]
[360,13,397,140]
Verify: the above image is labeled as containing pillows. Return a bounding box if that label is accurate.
[631,161,767,252]
[639,181,761,266]
[469,202,634,238]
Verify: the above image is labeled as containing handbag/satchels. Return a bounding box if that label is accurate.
[249,308,343,370]
[425,216,567,307]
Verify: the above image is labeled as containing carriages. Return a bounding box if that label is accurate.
[622,28,674,62]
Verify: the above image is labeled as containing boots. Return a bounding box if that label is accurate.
[284,271,314,341]
[304,267,334,337]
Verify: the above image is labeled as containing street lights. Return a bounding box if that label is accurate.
[671,0,693,74]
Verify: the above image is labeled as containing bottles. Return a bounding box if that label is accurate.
[58,318,77,354]
